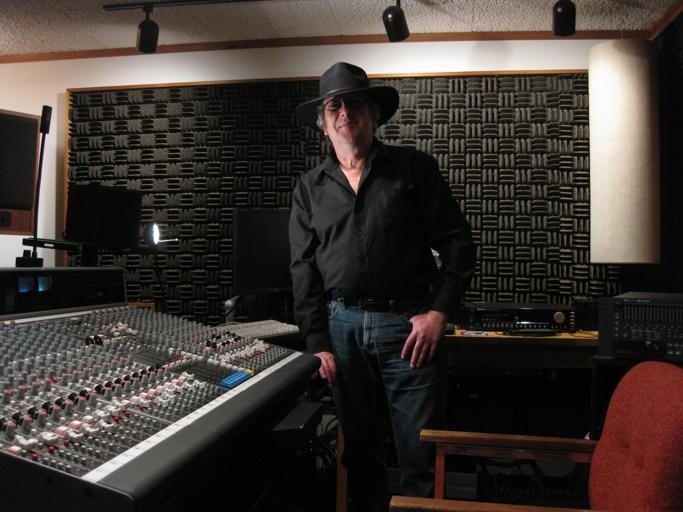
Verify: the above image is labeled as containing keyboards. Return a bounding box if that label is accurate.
[213,319,301,340]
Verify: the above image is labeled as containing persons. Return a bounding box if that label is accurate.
[285,60,478,509]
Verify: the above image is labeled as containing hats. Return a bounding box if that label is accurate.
[293,62,400,129]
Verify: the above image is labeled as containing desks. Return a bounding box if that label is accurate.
[431,323,603,441]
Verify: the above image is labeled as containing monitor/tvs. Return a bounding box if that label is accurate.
[232,206,292,294]
[63,183,144,254]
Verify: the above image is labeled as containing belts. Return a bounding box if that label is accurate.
[342,296,413,312]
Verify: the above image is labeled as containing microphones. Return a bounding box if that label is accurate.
[16,105,53,267]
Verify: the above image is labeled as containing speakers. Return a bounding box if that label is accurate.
[0,108,40,236]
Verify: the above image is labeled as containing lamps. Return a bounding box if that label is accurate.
[139,220,161,247]
[547,0,581,36]
[377,0,413,45]
[134,1,161,55]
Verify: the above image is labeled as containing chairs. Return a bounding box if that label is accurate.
[386,359,682,512]
